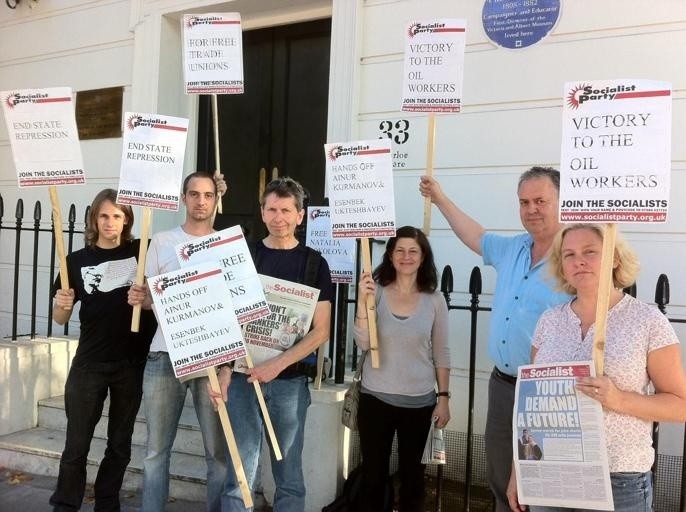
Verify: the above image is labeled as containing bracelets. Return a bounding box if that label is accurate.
[433,391,452,399]
[354,314,369,320]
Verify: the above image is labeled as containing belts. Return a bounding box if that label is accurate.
[493,363,516,387]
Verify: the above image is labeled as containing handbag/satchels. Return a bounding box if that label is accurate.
[341,350,367,430]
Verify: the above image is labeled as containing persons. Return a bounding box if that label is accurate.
[352,224,451,511]
[125,168,231,512]
[206,176,333,511]
[417,163,577,512]
[524,433,542,460]
[519,428,528,459]
[506,222,686,511]
[47,170,227,512]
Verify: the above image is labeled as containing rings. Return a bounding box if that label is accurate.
[594,388,599,395]
